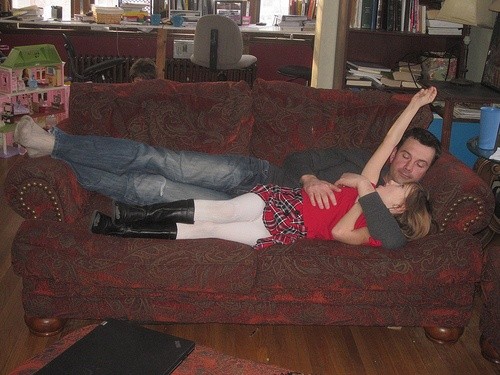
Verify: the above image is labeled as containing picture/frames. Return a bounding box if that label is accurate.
[215,1,242,25]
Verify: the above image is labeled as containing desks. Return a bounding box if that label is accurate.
[1,16,472,85]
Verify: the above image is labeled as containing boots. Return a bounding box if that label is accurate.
[112,198,195,225]
[88,209,178,241]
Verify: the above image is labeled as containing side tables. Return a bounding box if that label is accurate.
[467,136,500,295]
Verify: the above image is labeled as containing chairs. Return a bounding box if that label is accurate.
[190,14,258,84]
[273,37,314,87]
[62,33,125,84]
[417,15,500,153]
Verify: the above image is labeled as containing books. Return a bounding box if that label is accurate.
[346,59,423,89]
[279,0,317,34]
[74,0,260,25]
[453,106,482,119]
[349,0,463,35]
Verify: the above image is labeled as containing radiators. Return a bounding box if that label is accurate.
[66,55,256,84]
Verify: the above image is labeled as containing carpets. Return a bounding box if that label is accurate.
[1,323,305,375]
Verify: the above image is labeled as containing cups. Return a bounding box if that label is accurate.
[478,107,500,149]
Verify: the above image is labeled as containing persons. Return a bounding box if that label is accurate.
[89,86,437,250]
[130,56,157,83]
[14,115,442,248]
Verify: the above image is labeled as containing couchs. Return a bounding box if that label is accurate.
[6,78,496,345]
